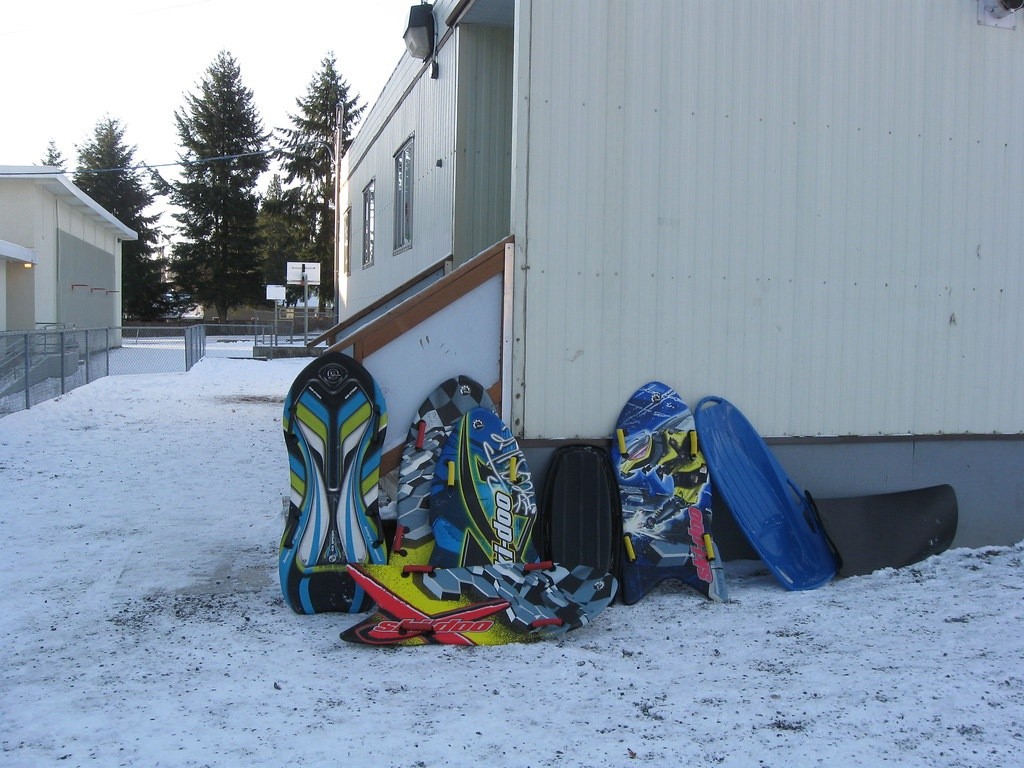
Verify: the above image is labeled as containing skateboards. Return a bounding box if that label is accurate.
[692,394,838,591]
[274,350,390,616]
[343,375,728,655]
